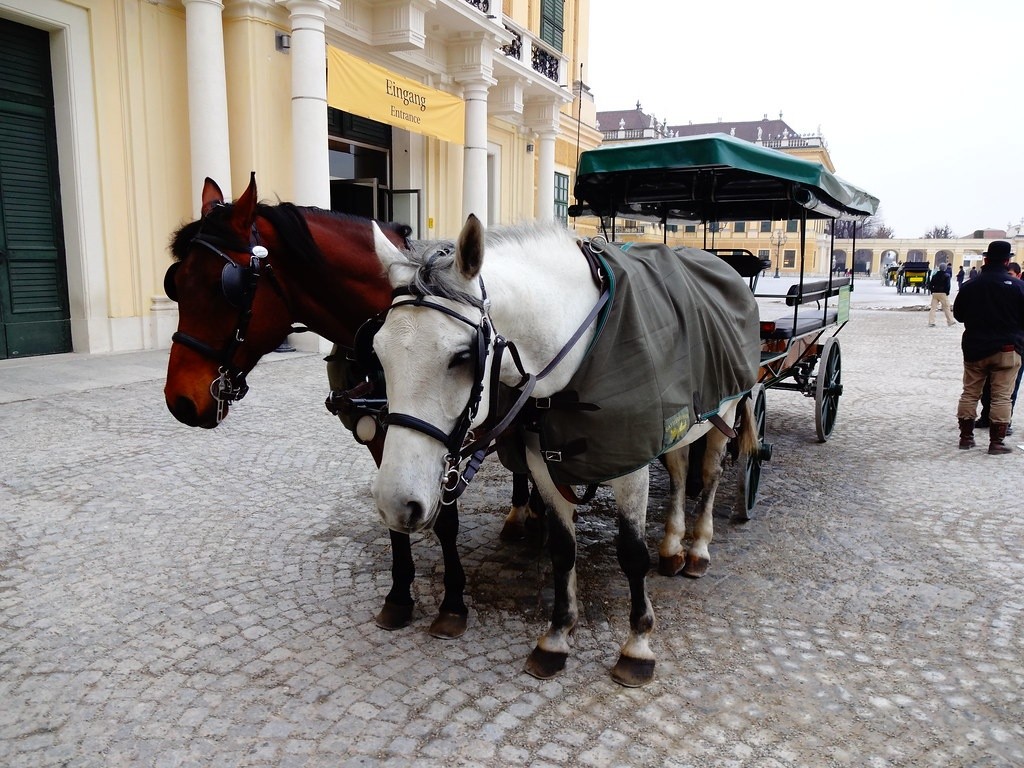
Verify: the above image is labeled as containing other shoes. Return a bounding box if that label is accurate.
[975,417,990,428]
[1005,423,1012,436]
[929,324,936,327]
[948,322,956,326]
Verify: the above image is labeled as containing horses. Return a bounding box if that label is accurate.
[165,170,760,689]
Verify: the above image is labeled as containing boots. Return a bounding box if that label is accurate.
[958,418,976,449]
[988,422,1012,455]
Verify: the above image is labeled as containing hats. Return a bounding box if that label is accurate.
[982,241,1015,258]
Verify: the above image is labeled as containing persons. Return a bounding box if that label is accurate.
[833,266,852,278]
[945,263,952,279]
[975,262,1024,436]
[928,263,956,328]
[969,266,977,279]
[952,241,1019,455]
[956,266,965,291]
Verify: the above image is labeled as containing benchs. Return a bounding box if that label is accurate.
[765,277,851,366]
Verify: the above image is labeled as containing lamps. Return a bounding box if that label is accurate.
[274,30,291,54]
[527,143,534,154]
